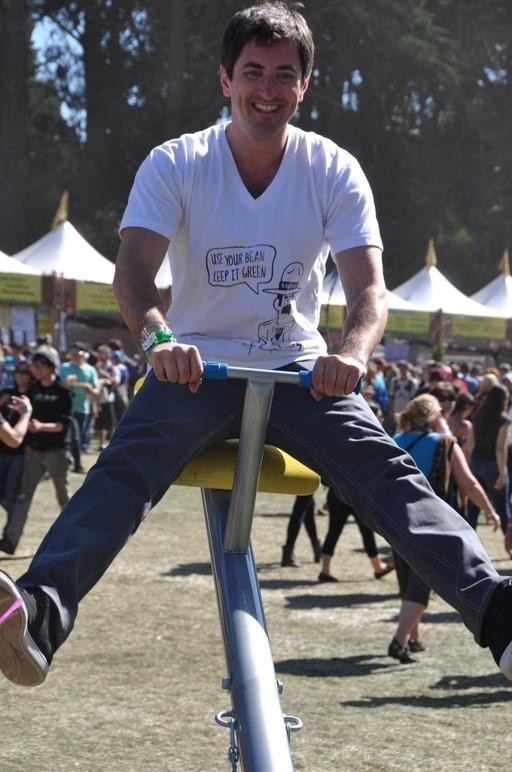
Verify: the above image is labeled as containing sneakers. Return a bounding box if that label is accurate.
[485,578,512,680]
[320,572,338,583]
[1,537,15,556]
[0,570,66,686]
[64,441,106,476]
[375,559,397,578]
[386,634,429,663]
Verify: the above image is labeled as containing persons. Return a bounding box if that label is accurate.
[279,339,512,581]
[1,330,146,563]
[388,393,502,666]
[1,1,512,684]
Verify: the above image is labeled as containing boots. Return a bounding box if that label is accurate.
[281,538,324,567]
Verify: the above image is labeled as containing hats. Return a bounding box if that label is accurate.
[374,358,510,392]
[2,336,121,367]
